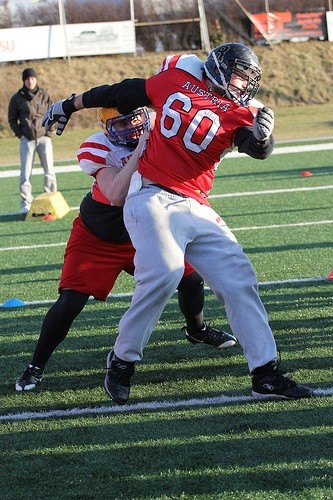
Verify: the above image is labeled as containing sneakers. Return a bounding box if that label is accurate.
[181,321,237,348]
[15,363,45,391]
[104,349,135,404]
[252,350,312,400]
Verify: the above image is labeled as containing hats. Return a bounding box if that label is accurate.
[22,68,37,81]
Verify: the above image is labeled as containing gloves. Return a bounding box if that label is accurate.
[253,105,274,141]
[42,93,78,135]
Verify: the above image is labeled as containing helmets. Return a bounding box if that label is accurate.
[204,43,262,107]
[97,103,151,149]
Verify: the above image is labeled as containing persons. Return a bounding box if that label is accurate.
[42,43,314,404]
[16,104,238,393]
[7,68,56,213]
